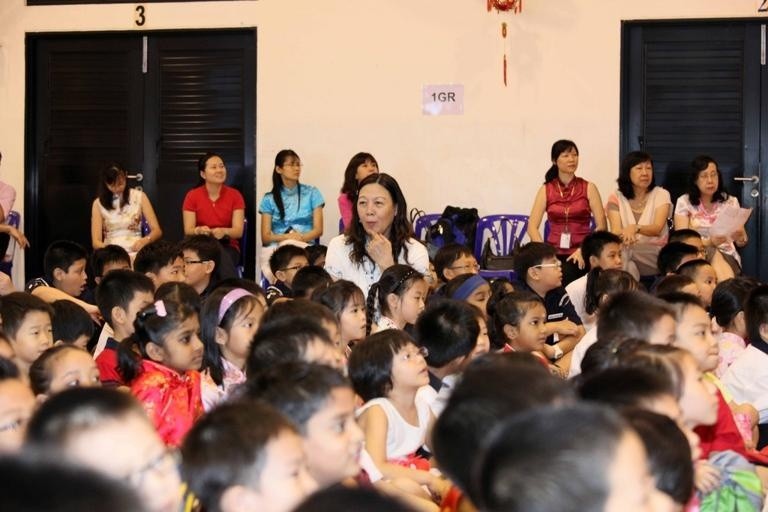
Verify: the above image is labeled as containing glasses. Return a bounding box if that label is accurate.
[449,263,481,272]
[280,264,304,272]
[283,162,303,167]
[119,443,185,485]
[393,346,430,363]
[1,418,28,433]
[531,260,562,270]
[184,259,208,266]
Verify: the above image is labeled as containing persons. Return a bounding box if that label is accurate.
[0,229,768,510]
[672,156,748,279]
[180,152,245,282]
[322,172,430,301]
[0,150,28,296]
[526,140,606,270]
[88,166,163,252]
[336,151,378,231]
[258,148,325,284]
[606,151,673,274]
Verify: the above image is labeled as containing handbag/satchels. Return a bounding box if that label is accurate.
[481,218,522,270]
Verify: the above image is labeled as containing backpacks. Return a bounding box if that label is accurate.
[426,205,480,262]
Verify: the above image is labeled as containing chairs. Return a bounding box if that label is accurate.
[476,214,531,279]
[543,216,596,244]
[415,213,441,240]
